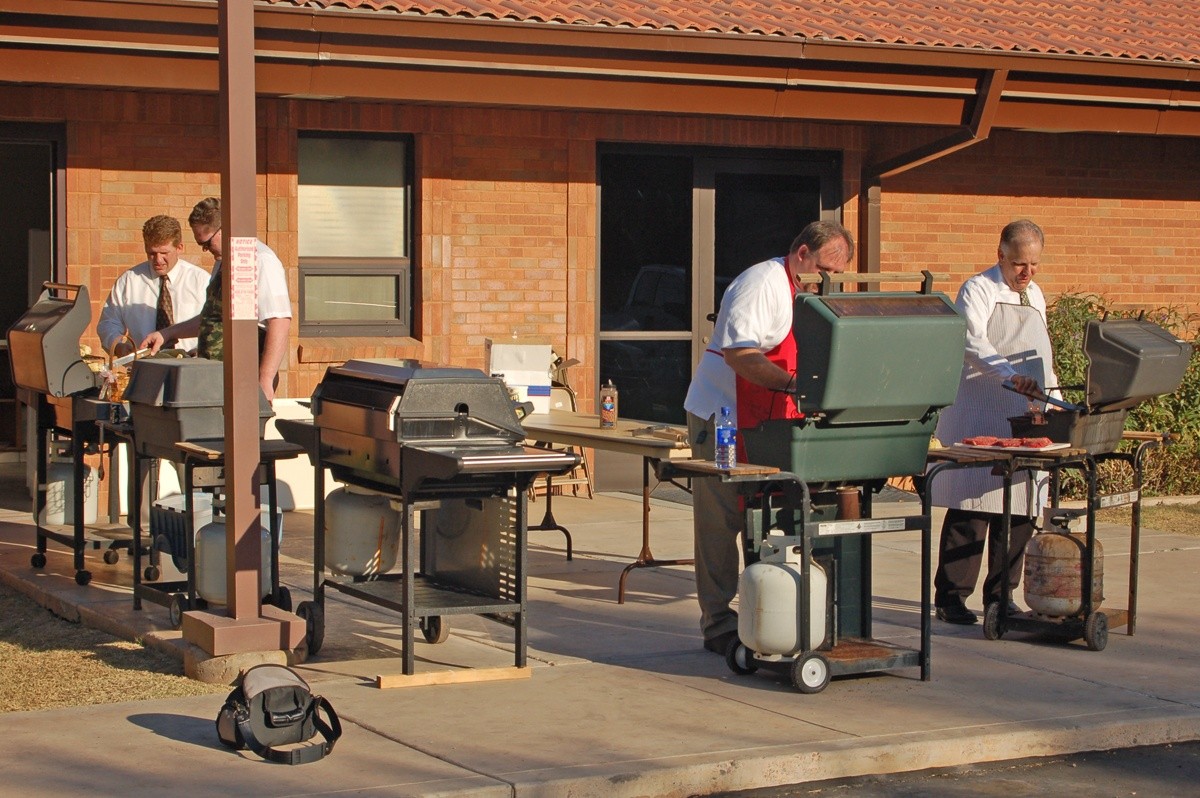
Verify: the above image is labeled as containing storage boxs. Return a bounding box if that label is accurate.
[484,337,581,414]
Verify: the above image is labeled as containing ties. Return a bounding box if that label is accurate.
[156,276,179,350]
[1019,290,1030,308]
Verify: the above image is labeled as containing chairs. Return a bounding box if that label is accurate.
[526,387,594,502]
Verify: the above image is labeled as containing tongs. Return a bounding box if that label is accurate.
[1001,379,1085,410]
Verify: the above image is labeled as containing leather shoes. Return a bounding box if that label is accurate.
[985,599,1023,616]
[934,602,978,624]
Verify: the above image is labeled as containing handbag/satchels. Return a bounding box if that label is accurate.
[215,662,342,765]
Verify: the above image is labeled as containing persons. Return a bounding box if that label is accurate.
[97,215,211,556]
[933,218,1065,625]
[140,197,292,409]
[684,221,856,656]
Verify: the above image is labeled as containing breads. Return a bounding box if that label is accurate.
[80,344,100,372]
[148,348,192,358]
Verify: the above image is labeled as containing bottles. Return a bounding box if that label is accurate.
[714,406,737,469]
[599,385,618,429]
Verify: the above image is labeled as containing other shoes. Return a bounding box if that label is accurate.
[703,630,740,654]
[128,546,151,555]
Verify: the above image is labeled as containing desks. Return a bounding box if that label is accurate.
[519,409,694,604]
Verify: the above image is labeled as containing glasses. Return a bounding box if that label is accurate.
[198,226,222,249]
